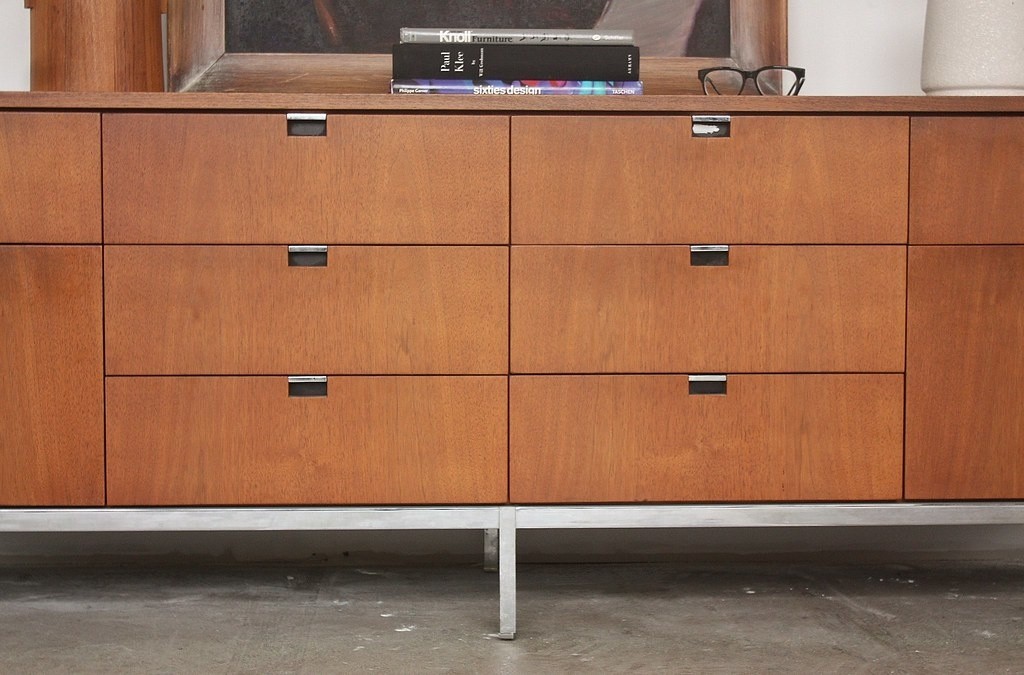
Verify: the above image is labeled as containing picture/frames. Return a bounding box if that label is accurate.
[167,0,789,98]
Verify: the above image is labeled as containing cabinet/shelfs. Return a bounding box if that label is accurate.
[0,93,1024,640]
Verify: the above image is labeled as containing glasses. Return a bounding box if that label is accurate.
[698,65,807,96]
